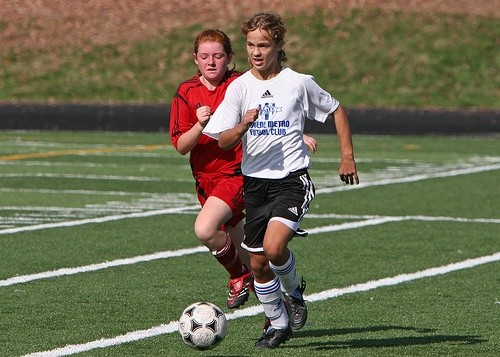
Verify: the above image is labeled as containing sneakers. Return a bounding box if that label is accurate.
[282,276,308,331]
[226,263,253,309]
[263,298,289,337]
[253,322,293,351]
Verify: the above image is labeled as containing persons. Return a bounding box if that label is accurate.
[202,11,361,348]
[170,30,318,335]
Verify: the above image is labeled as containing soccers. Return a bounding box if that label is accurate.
[178,302,228,351]
